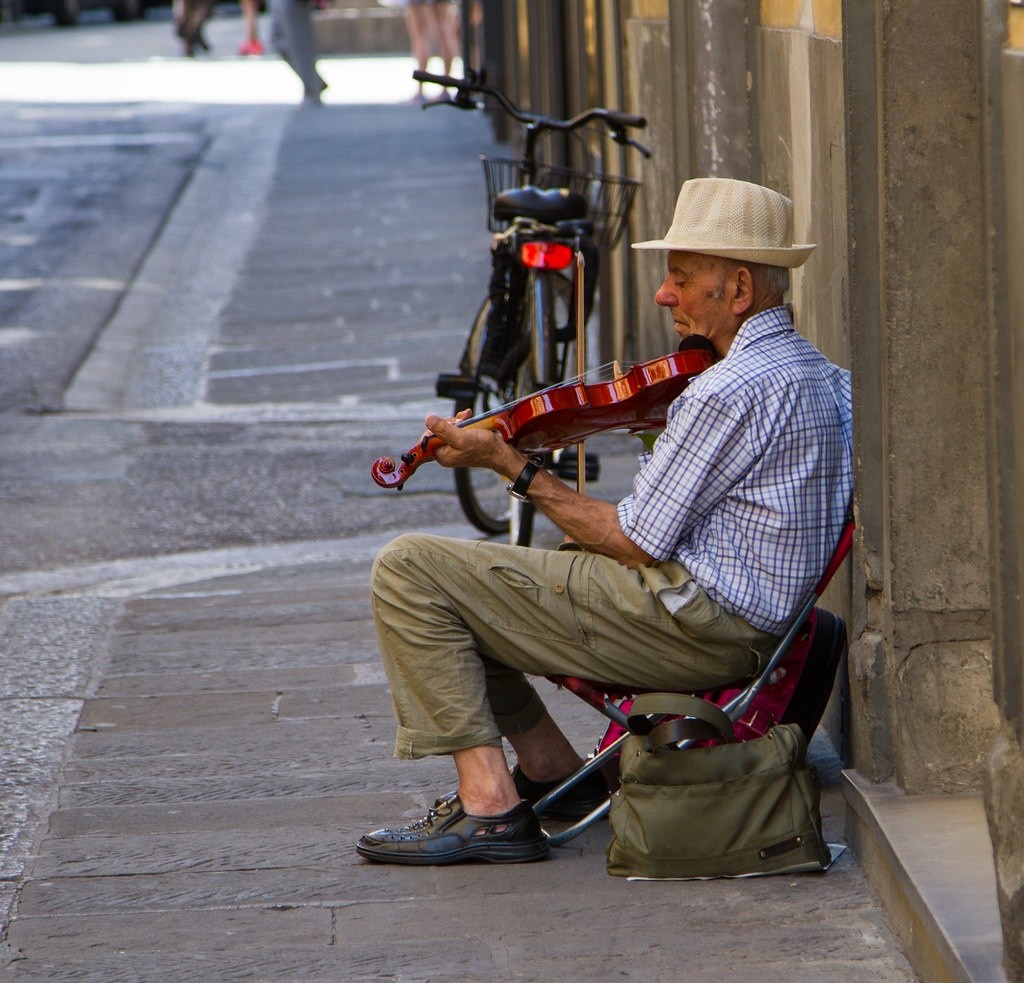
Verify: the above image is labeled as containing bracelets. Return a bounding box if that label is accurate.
[507,456,545,503]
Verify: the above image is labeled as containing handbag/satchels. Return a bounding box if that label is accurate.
[605,694,832,877]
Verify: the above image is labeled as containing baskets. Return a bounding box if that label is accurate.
[482,154,639,257]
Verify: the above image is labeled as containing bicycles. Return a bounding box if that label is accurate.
[390,63,656,551]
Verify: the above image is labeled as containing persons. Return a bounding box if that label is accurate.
[357,178,852,866]
[237,0,261,55]
[172,0,211,55]
[405,0,461,103]
[268,0,327,104]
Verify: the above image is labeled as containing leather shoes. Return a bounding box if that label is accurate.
[356,797,551,863]
[429,763,612,821]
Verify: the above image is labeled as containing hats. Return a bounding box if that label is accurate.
[631,178,817,268]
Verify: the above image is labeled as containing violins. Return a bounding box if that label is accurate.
[372,348,716,490]
